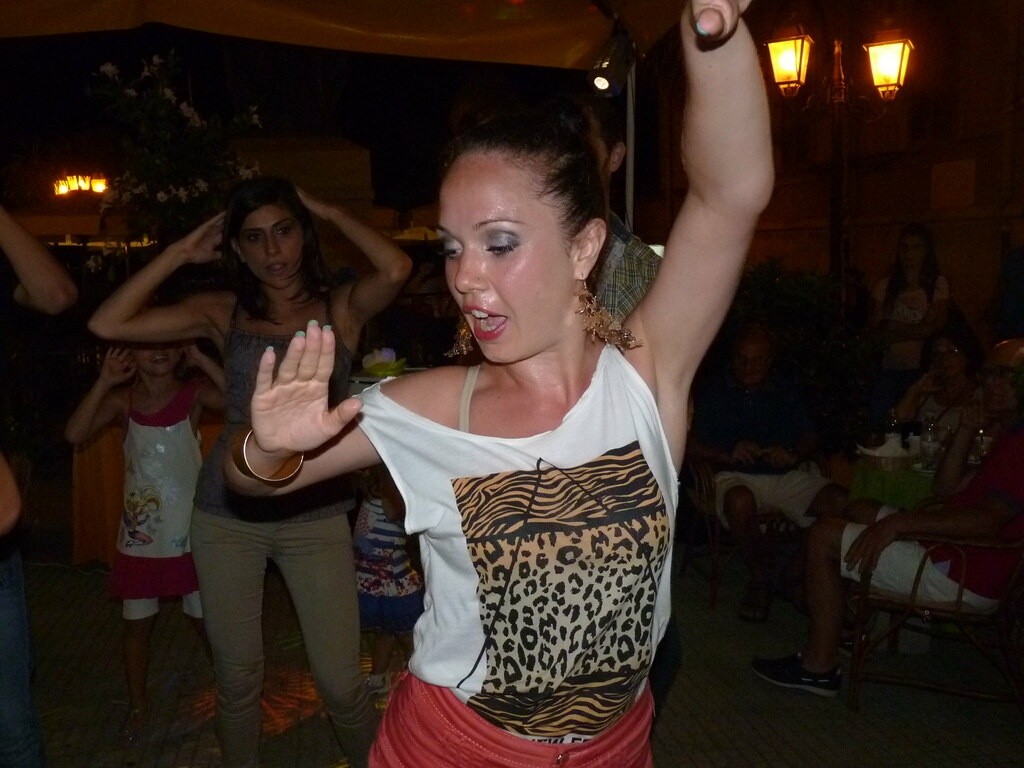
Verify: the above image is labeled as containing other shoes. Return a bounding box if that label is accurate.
[112,712,144,752]
[780,585,809,615]
[738,580,779,621]
[364,673,391,696]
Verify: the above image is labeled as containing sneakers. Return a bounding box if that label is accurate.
[838,631,867,656]
[749,651,841,698]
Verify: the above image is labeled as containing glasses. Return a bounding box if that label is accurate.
[974,364,1016,387]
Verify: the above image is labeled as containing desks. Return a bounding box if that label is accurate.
[70,408,224,571]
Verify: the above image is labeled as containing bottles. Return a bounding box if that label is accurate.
[883,408,901,448]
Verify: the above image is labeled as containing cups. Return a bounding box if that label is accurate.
[921,421,939,466]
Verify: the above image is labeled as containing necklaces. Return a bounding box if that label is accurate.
[919,379,969,424]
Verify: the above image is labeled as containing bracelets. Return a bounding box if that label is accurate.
[230,425,304,484]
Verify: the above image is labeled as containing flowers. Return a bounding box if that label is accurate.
[361,347,408,378]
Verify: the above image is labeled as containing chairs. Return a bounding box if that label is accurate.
[846,536,1024,713]
[686,452,834,611]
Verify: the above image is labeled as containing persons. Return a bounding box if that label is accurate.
[223,0,776,768]
[0,86,1024,768]
